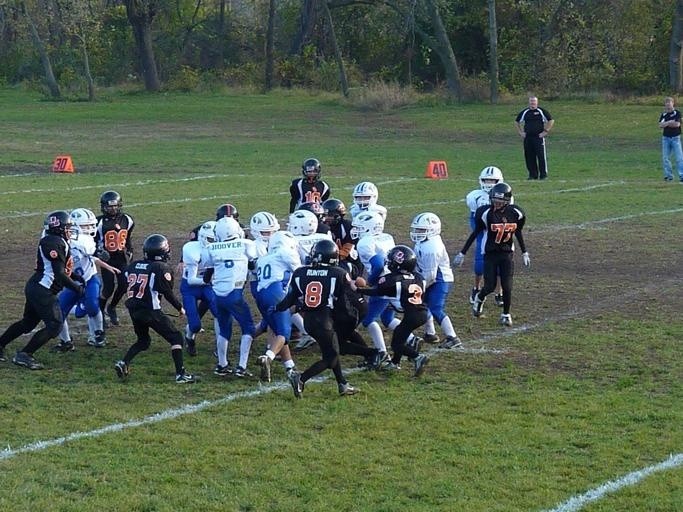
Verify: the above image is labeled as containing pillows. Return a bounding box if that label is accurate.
[101,190,124,215]
[302,158,321,180]
[288,210,318,235]
[48,210,79,242]
[488,183,511,207]
[213,218,245,241]
[352,181,379,210]
[142,234,171,263]
[323,198,346,221]
[478,166,503,192]
[386,246,417,273]
[310,239,339,265]
[300,202,325,225]
[216,204,240,223]
[409,212,441,244]
[70,208,98,237]
[349,211,384,240]
[268,231,299,252]
[198,221,217,246]
[250,212,279,240]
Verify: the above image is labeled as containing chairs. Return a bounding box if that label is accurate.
[371,351,387,367]
[70,305,76,314]
[290,334,317,353]
[500,313,512,325]
[473,298,485,317]
[437,335,461,350]
[407,337,423,362]
[380,355,392,367]
[338,382,360,396]
[289,334,300,342]
[12,352,44,370]
[89,335,94,344]
[107,306,120,325]
[663,177,673,180]
[257,354,273,383]
[494,294,504,306]
[214,360,236,375]
[678,176,682,183]
[286,366,306,378]
[200,328,204,332]
[470,287,480,305]
[412,356,430,377]
[91,330,106,347]
[540,174,548,180]
[1,346,8,361]
[186,336,198,356]
[213,351,218,357]
[48,340,74,353]
[234,366,256,379]
[116,359,130,382]
[290,375,305,400]
[175,370,195,384]
[527,176,537,180]
[423,333,440,343]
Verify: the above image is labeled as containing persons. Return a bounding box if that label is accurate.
[409,212,462,349]
[287,212,334,350]
[351,246,431,377]
[351,212,425,367]
[658,97,683,182]
[467,166,514,306]
[202,216,254,378]
[267,241,360,399]
[298,202,330,238]
[289,158,330,216]
[177,205,248,333]
[515,96,554,180]
[116,235,203,385]
[240,212,280,355]
[42,225,107,356]
[97,192,136,329]
[181,220,220,360]
[1,211,84,371]
[322,199,368,286]
[348,183,387,225]
[453,185,530,325]
[67,209,121,318]
[334,251,387,369]
[257,231,302,384]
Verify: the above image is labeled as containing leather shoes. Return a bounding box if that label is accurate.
[128,252,134,260]
[522,252,532,269]
[268,305,281,315]
[99,251,111,262]
[80,278,88,288]
[454,252,465,267]
[77,287,85,298]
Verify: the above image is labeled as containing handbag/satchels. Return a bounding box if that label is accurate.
[544,129,549,133]
